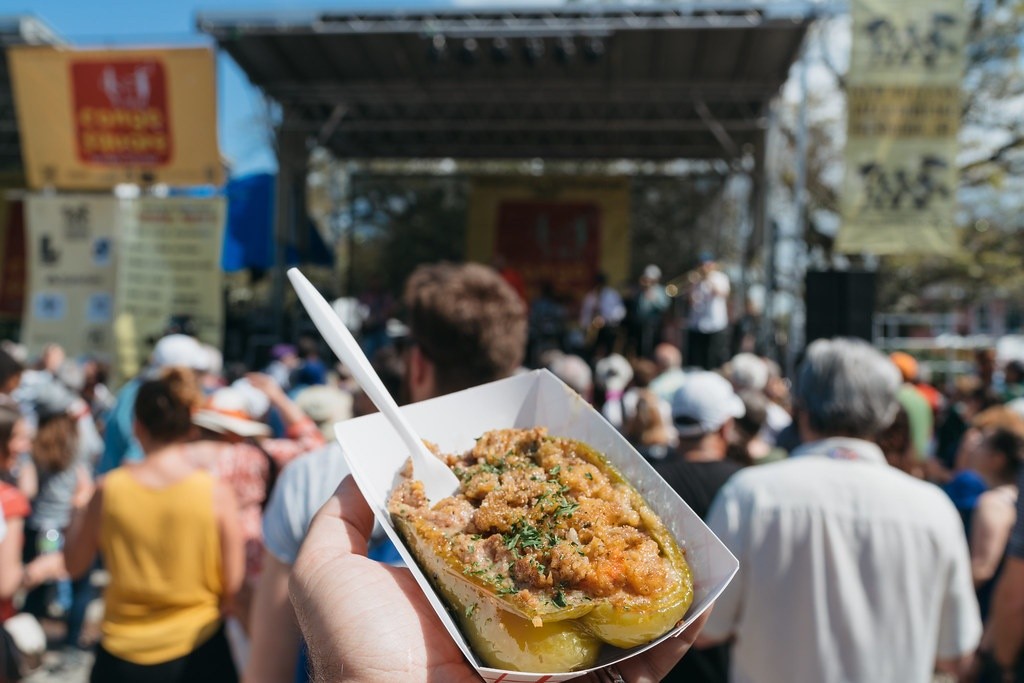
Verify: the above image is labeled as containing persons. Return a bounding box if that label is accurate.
[0,241,1022,682]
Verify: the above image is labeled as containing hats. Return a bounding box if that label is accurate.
[191,387,273,437]
[672,371,746,436]
[891,351,920,381]
[140,334,210,381]
[291,358,327,387]
[231,378,271,419]
[297,386,355,441]
